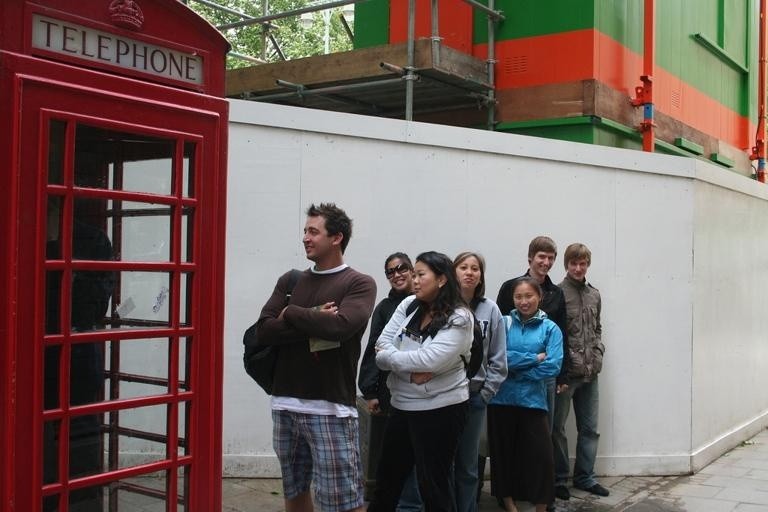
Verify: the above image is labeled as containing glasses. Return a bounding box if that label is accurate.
[385,263,409,279]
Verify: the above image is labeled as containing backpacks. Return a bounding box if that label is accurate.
[406,298,484,379]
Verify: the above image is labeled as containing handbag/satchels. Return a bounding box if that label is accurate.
[242,320,276,396]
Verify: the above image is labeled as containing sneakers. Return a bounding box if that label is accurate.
[555,486,569,500]
[588,484,609,496]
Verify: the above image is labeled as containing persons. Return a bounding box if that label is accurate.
[551,242,610,500]
[257,203,378,512]
[373,253,475,512]
[46,174,117,510]
[485,275,565,512]
[447,250,510,512]
[489,236,574,511]
[357,253,416,512]
